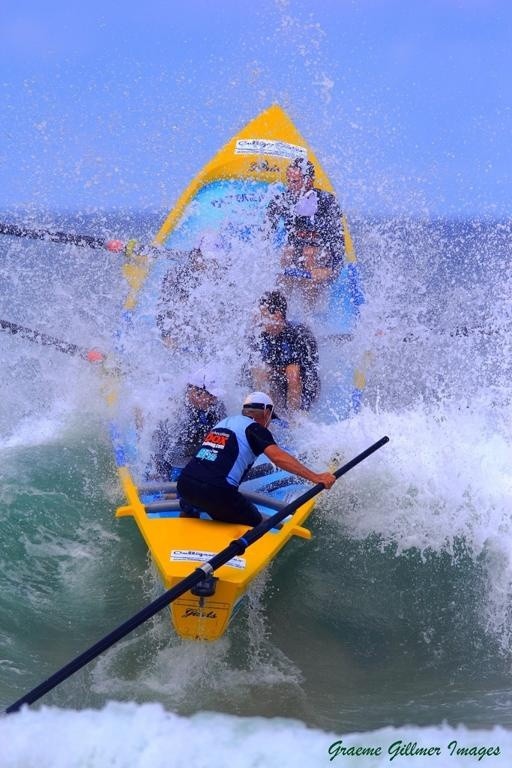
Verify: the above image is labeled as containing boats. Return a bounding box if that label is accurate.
[103,103,369,644]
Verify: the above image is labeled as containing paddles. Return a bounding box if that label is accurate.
[5,438,388,711]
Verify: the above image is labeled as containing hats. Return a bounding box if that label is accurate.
[188,367,227,397]
[242,392,281,421]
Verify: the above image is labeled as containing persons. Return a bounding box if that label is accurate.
[242,286,321,451]
[178,390,336,527]
[257,158,344,318]
[153,228,245,372]
[143,370,224,498]
